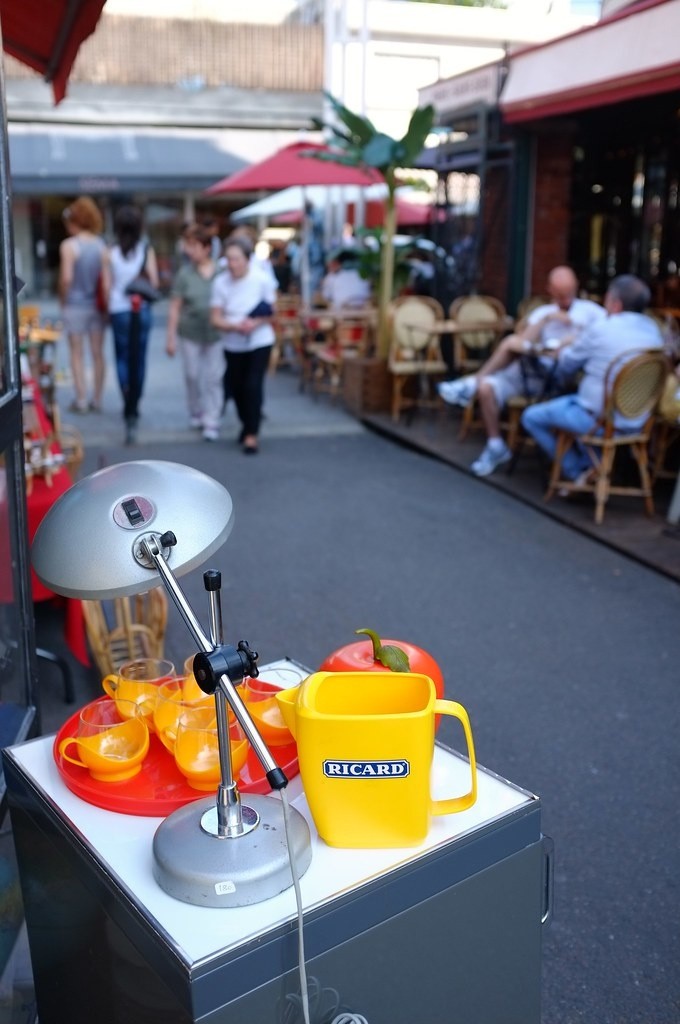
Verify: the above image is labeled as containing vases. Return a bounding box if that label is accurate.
[299,87,439,417]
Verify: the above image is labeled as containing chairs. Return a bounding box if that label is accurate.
[271,292,680,525]
[16,308,166,681]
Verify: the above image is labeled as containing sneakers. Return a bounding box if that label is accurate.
[472,441,512,477]
[439,373,477,406]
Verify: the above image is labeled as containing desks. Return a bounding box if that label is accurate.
[0,375,92,670]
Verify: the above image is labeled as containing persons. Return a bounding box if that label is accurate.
[210,240,276,451]
[322,251,367,310]
[519,275,664,495]
[165,225,227,442]
[106,204,159,426]
[59,197,113,416]
[170,222,261,279]
[437,266,604,476]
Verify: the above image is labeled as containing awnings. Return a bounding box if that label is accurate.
[8,132,252,194]
[0,0,107,108]
[498,0,680,119]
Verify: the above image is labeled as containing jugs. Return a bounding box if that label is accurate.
[275,671,477,849]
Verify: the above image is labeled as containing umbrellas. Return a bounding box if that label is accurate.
[203,141,444,308]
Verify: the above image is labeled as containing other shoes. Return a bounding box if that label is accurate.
[200,416,221,441]
[124,417,138,443]
[86,400,102,415]
[243,438,259,455]
[189,410,203,428]
[559,469,598,498]
[70,400,88,413]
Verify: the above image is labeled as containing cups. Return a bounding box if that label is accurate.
[102,658,179,733]
[173,707,250,791]
[245,669,302,746]
[58,699,149,782]
[154,677,215,754]
[183,652,250,708]
